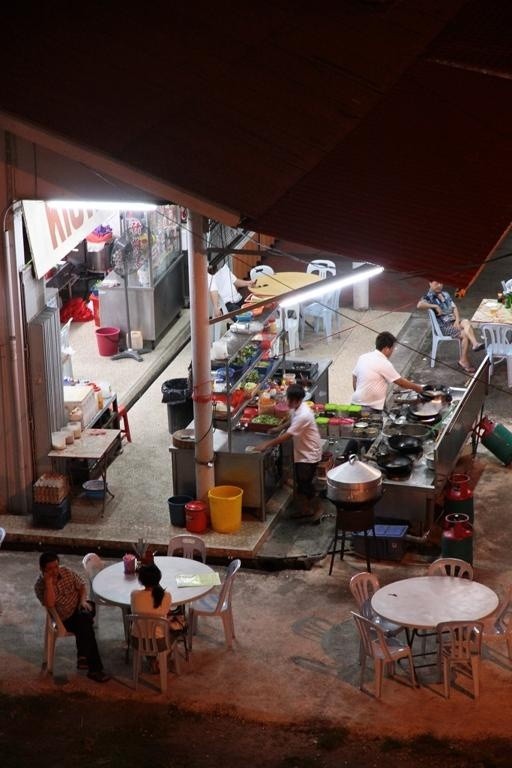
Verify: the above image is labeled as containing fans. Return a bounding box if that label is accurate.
[111,225,157,362]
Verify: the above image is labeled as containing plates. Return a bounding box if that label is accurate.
[353,421,369,427]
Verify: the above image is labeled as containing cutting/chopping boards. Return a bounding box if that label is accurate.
[172,429,195,449]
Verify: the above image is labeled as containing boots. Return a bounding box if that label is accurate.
[311,493,326,524]
[291,496,313,518]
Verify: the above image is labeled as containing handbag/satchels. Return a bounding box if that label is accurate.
[168,604,187,635]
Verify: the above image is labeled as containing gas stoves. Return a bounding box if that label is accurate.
[276,360,318,378]
[359,355,489,541]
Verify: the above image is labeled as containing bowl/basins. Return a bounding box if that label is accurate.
[381,423,432,445]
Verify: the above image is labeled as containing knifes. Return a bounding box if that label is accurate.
[181,435,196,441]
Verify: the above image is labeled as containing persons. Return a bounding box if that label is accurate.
[255,384,326,522]
[345,331,423,456]
[130,564,173,674]
[417,275,485,373]
[34,551,112,683]
[209,264,256,323]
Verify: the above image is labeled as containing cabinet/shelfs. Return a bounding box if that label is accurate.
[95,251,185,350]
[362,382,477,537]
[169,298,333,522]
[85,384,120,430]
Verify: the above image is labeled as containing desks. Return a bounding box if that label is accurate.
[92,556,216,664]
[247,272,320,297]
[370,574,500,688]
[471,298,512,328]
[47,428,121,518]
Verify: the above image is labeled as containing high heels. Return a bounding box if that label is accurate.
[150,661,156,673]
[156,659,169,672]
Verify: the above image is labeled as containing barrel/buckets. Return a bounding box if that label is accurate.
[96,326,120,356]
[209,485,243,534]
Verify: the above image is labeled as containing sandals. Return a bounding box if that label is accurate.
[78,658,89,669]
[88,669,109,681]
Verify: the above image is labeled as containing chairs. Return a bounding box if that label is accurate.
[81,552,130,642]
[166,534,206,564]
[186,560,241,651]
[427,308,462,368]
[420,558,473,658]
[481,323,512,388]
[42,601,99,675]
[249,265,274,280]
[350,610,416,701]
[300,289,342,343]
[435,620,485,702]
[306,259,336,279]
[475,589,512,664]
[124,614,180,695]
[349,572,411,676]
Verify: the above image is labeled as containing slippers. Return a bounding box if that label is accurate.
[472,342,484,352]
[457,360,476,372]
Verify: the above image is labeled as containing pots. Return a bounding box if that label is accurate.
[387,434,422,454]
[407,401,439,420]
[420,384,453,400]
[363,452,412,472]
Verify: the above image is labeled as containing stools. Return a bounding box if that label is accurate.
[110,404,131,442]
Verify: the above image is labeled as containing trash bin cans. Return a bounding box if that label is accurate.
[161,378,195,434]
